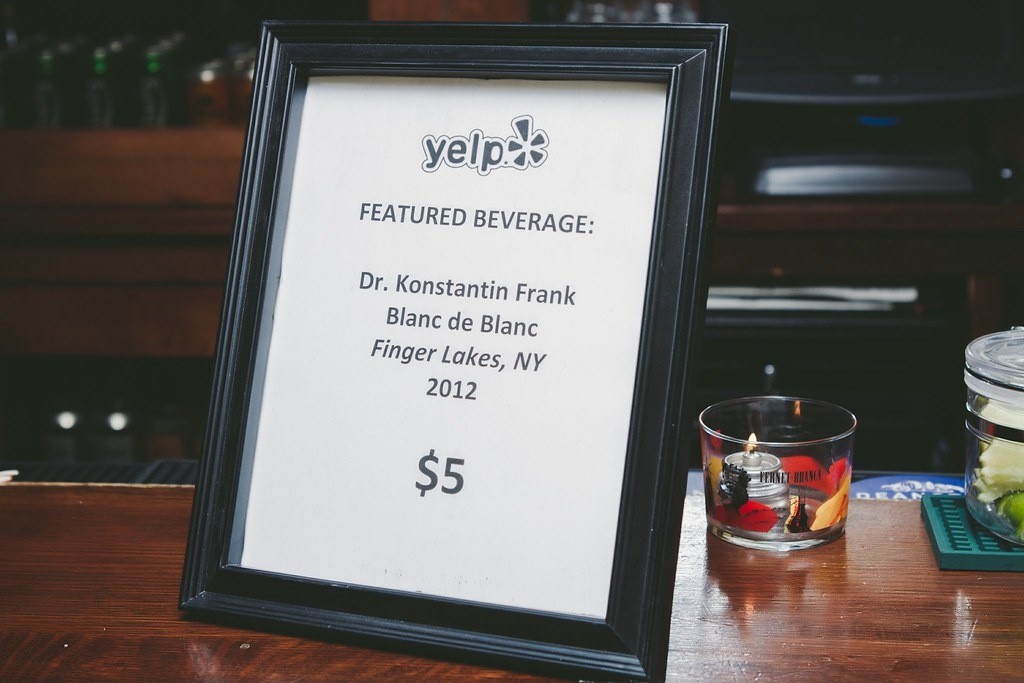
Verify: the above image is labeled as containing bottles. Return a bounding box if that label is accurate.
[3,25,187,132]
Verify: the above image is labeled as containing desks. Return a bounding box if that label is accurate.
[1,482,1024,683]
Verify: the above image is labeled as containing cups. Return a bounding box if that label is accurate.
[699,397,856,551]
[963,326,1024,547]
